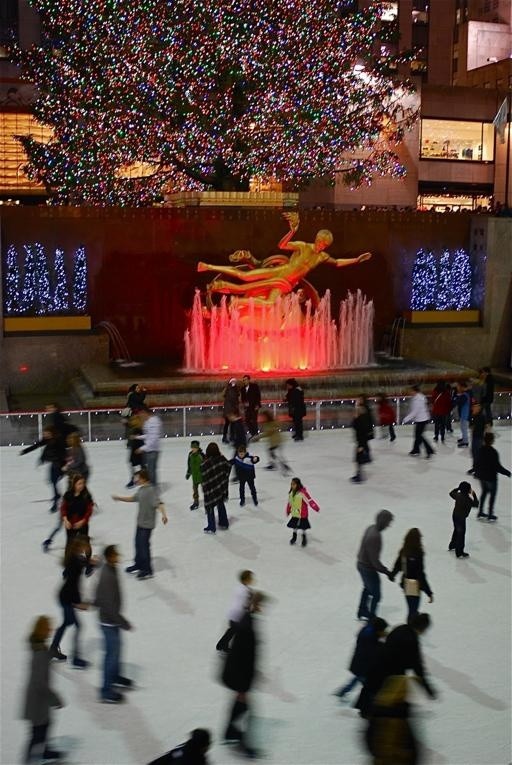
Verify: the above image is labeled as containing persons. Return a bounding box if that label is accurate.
[197,212,372,298]
[335,612,439,765]
[286,477,320,547]
[350,366,496,484]
[448,481,480,559]
[185,374,307,534]
[473,432,512,522]
[356,510,433,626]
[17,534,135,764]
[148,728,214,765]
[214,569,272,760]
[17,383,169,579]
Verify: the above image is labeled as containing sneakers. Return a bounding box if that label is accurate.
[126,565,153,577]
[43,495,61,553]
[52,653,131,703]
[186,460,308,548]
[347,438,500,560]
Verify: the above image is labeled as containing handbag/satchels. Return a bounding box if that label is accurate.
[404,578,419,597]
